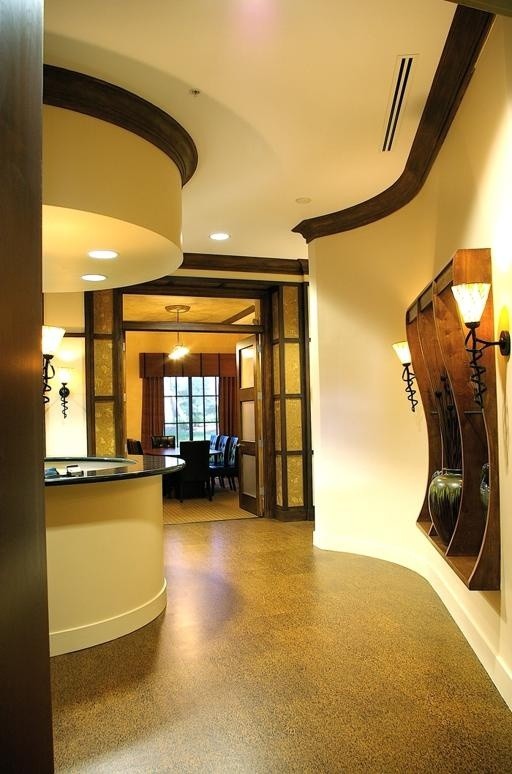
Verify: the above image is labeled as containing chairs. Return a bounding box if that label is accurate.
[127,435,239,504]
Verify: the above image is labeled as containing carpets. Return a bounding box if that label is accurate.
[163,486,259,525]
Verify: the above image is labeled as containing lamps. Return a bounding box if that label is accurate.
[41,325,70,419]
[450,282,510,409]
[393,342,418,413]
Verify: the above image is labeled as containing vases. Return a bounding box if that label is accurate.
[428,467,462,547]
